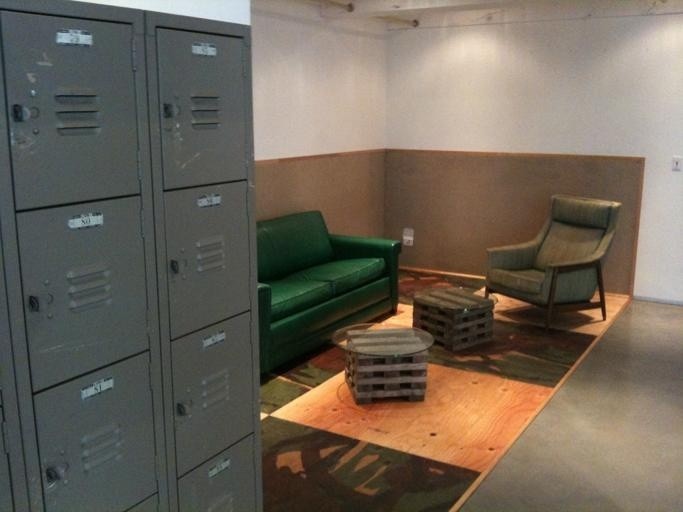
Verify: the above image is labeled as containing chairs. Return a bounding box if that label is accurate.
[484,194,623,332]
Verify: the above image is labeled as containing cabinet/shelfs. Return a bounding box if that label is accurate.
[0,5,265,511]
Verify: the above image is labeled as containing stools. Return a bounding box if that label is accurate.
[413,287,495,354]
[345,329,430,405]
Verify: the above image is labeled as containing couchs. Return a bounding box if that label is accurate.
[256,209,401,383]
